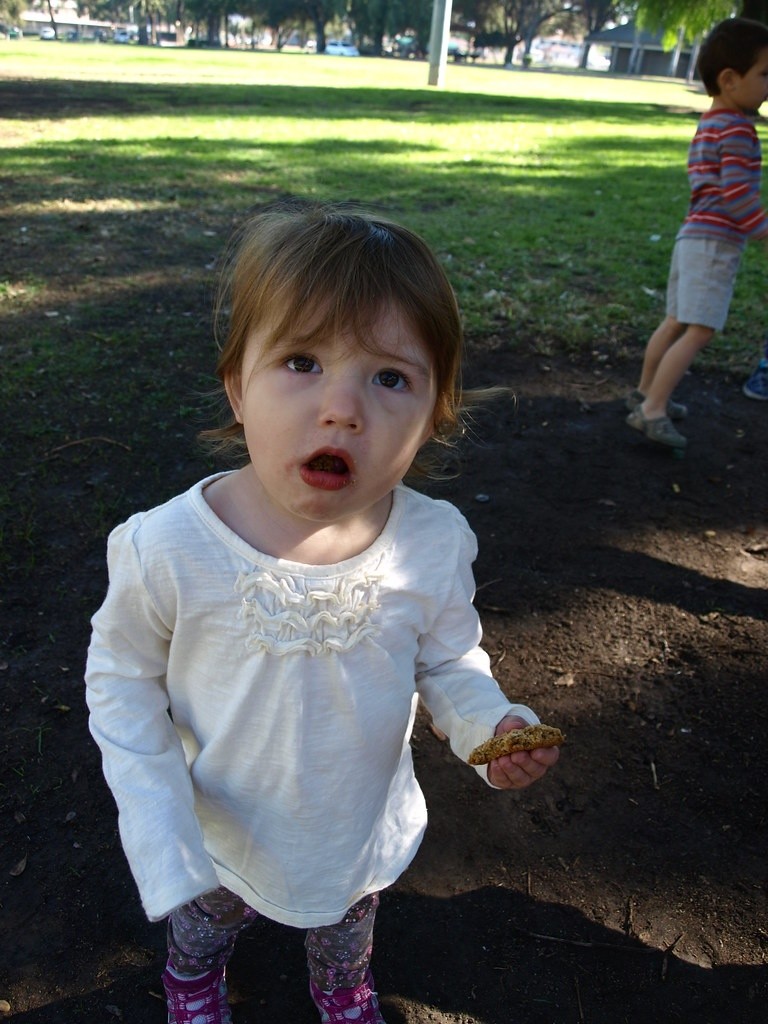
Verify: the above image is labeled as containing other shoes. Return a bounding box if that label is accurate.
[626,403,685,446]
[743,359,768,400]
[625,371,693,411]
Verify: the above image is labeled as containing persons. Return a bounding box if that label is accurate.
[624,19,768,448]
[84,210,561,1024]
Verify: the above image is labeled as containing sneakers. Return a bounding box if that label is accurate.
[163,953,232,1024]
[311,967,385,1024]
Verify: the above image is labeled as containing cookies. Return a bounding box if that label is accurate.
[469,723,565,764]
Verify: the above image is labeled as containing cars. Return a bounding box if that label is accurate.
[424,35,469,62]
[0,23,23,39]
[40,26,56,40]
[391,34,419,58]
[114,30,129,43]
[93,28,107,41]
[325,39,359,56]
